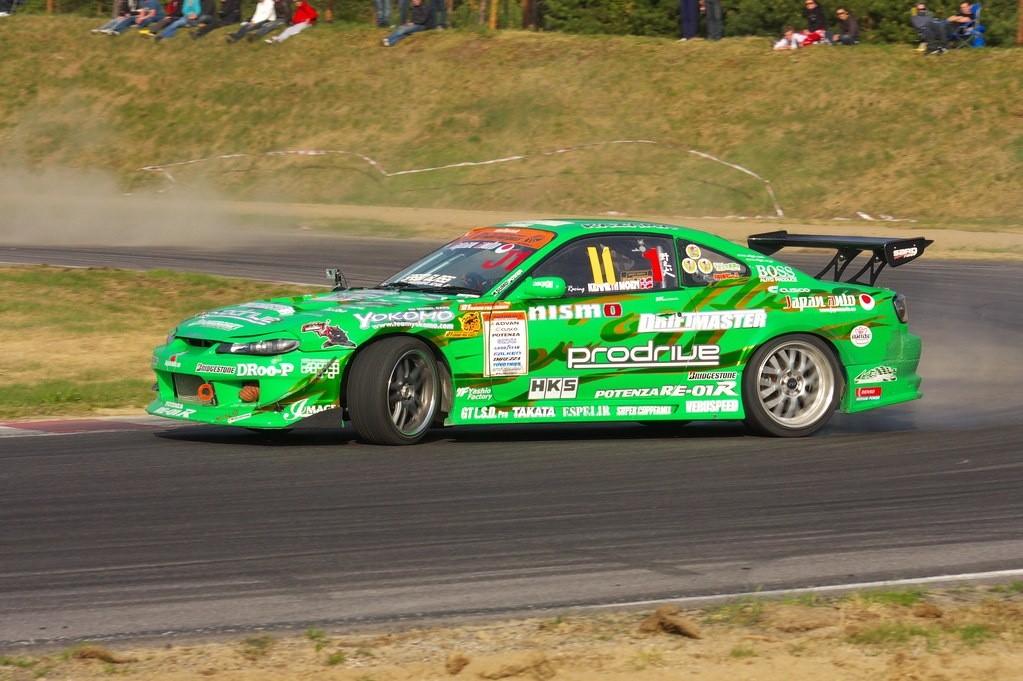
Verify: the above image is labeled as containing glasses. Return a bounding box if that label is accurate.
[836,11,844,15]
[805,1,814,4]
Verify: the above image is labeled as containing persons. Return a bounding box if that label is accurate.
[678,0,699,42]
[910,1,975,53]
[700,0,724,40]
[374,0,448,47]
[224,0,318,44]
[773,0,860,50]
[0,0,16,17]
[89,0,242,42]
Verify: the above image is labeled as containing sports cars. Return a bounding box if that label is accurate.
[146,218,934,448]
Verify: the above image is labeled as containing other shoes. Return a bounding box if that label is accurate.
[108,31,121,35]
[913,47,928,54]
[380,37,390,46]
[100,28,111,33]
[222,31,237,42]
[90,28,100,34]
